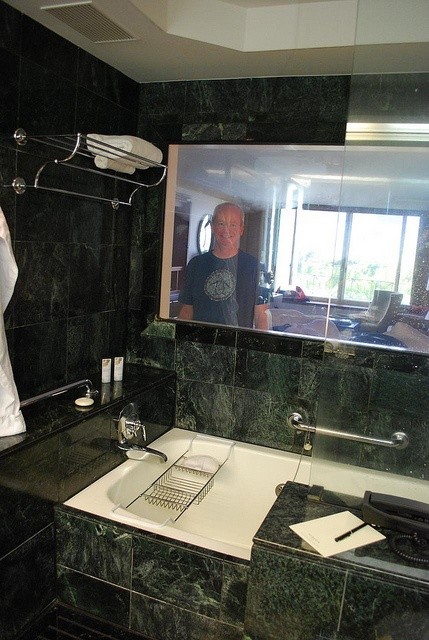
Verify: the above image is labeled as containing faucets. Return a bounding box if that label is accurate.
[113,400,168,464]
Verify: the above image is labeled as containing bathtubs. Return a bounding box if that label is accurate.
[63,427,429,563]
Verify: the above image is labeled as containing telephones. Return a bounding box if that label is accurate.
[363,491,429,563]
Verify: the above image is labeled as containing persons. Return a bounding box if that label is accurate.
[179,203,272,332]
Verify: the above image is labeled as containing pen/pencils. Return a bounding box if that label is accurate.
[336,522,369,542]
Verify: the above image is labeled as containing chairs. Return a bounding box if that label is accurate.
[362,293,404,333]
[348,290,392,322]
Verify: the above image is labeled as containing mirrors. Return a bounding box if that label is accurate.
[157,143,429,355]
[196,213,211,254]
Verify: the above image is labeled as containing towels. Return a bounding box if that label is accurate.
[83,131,163,176]
[0,205,27,438]
